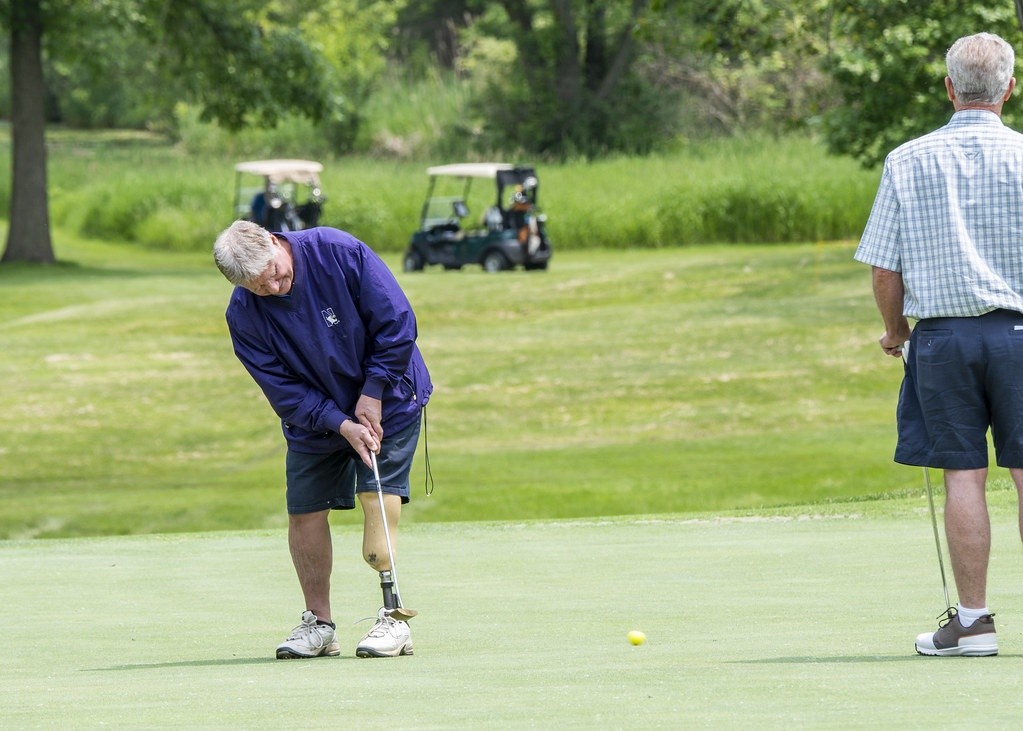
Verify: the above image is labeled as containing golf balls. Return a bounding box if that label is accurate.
[628,631,646,645]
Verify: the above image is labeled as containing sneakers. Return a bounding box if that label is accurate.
[914,607,999,656]
[352,607,414,657]
[276,610,340,659]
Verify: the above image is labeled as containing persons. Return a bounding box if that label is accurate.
[252,179,279,222]
[214,220,434,659]
[854,32,1023,658]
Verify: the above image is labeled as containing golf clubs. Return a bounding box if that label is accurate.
[366,430,418,621]
[897,347,951,618]
